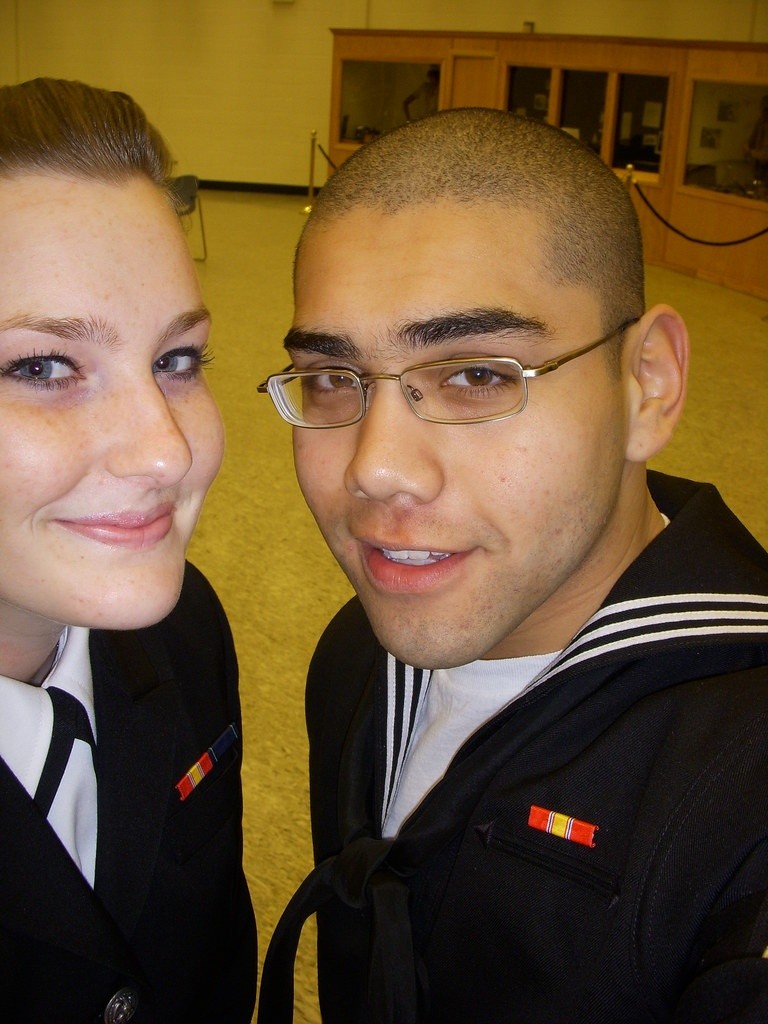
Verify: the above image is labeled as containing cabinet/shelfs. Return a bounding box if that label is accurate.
[326,26,768,299]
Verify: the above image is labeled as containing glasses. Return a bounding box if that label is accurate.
[256,316,643,430]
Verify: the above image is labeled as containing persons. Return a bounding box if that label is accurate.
[259,105,768,1024]
[0,75,256,1023]
